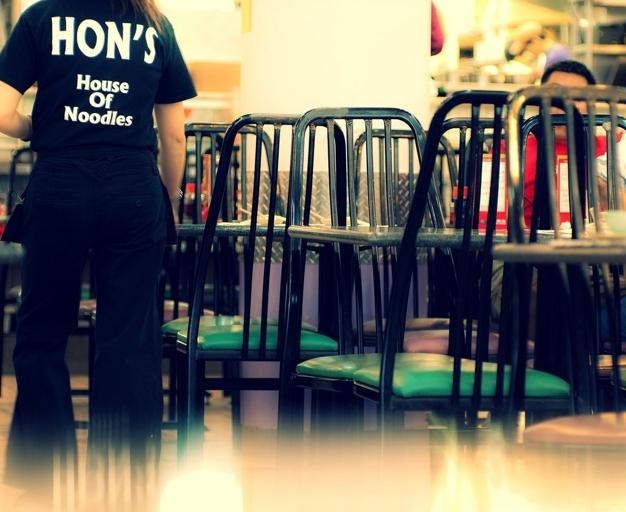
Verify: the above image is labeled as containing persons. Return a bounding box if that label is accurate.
[476,59,626,418]
[593,132,626,344]
[1,0,198,495]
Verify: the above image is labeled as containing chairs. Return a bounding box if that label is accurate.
[1,142,95,433]
[151,85,625,485]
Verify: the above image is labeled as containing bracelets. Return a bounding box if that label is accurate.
[20,113,34,144]
[168,188,185,204]
